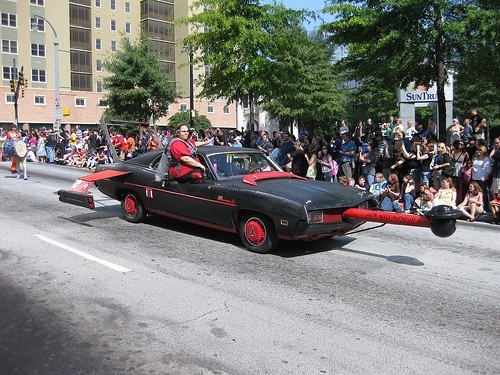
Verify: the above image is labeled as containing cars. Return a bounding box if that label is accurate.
[54,107,463,254]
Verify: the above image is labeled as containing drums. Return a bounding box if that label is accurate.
[1,139,27,158]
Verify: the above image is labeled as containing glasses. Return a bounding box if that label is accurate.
[177,129,188,132]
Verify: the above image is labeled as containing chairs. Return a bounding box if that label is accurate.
[218,157,241,176]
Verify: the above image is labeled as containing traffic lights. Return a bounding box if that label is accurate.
[21,89,24,97]
[19,71,24,86]
[24,79,28,88]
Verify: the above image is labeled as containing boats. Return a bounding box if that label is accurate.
[10,79,15,92]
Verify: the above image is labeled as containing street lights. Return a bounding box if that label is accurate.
[181,36,194,132]
[32,14,61,144]
